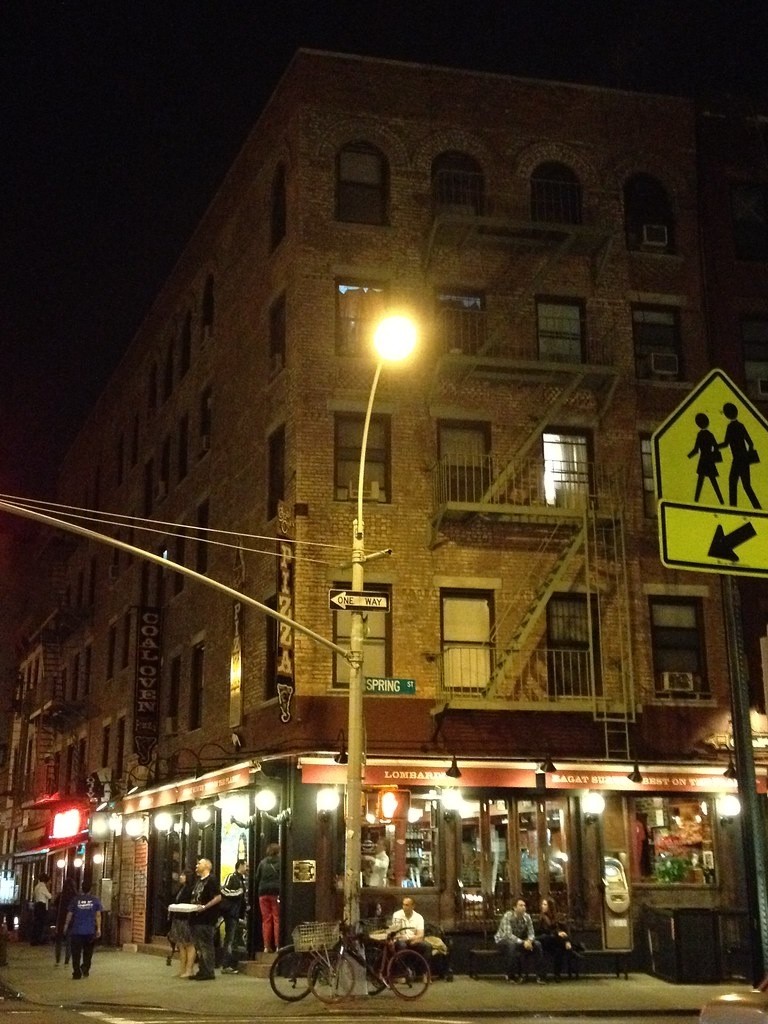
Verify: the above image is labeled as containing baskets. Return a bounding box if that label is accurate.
[292,922,341,952]
[364,916,406,940]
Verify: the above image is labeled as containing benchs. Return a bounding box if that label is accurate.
[366,925,455,982]
[166,925,223,967]
[469,920,582,979]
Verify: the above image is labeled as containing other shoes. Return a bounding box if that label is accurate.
[536,975,545,984]
[554,973,562,982]
[263,947,274,953]
[402,971,417,983]
[172,969,185,977]
[423,973,432,984]
[510,975,523,983]
[72,973,83,980]
[180,970,194,978]
[574,952,585,961]
[80,965,89,978]
[221,966,239,973]
[189,972,215,980]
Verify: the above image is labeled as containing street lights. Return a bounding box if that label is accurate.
[342,302,427,995]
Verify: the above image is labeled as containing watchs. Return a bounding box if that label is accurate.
[201,905,206,911]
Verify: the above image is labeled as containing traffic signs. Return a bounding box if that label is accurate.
[328,588,391,613]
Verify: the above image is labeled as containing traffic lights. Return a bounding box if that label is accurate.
[377,789,412,823]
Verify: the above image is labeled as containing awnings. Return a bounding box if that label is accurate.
[13,834,87,865]
[122,760,250,815]
[297,757,768,794]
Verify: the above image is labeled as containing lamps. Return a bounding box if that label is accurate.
[711,743,736,779]
[442,788,461,820]
[718,795,742,825]
[335,729,350,765]
[316,787,340,824]
[582,791,605,824]
[627,747,642,783]
[94,742,293,843]
[441,747,462,777]
[540,749,559,773]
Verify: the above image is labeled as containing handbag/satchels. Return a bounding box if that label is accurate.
[216,896,241,918]
[40,918,58,946]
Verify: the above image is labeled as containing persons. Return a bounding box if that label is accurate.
[168,868,198,979]
[367,900,383,917]
[362,837,390,888]
[63,880,103,979]
[538,897,571,982]
[255,843,281,953]
[220,859,249,974]
[188,858,222,981]
[392,896,432,984]
[54,878,79,966]
[420,867,434,886]
[495,897,547,986]
[29,873,52,946]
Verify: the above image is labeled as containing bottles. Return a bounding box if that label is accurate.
[404,824,423,858]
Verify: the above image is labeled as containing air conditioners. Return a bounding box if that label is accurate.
[348,479,380,501]
[754,377,768,395]
[644,352,679,376]
[640,223,668,247]
[656,671,695,693]
[267,354,282,378]
[151,481,166,502]
[102,563,119,584]
[198,434,211,456]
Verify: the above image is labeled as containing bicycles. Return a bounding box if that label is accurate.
[268,916,432,1004]
